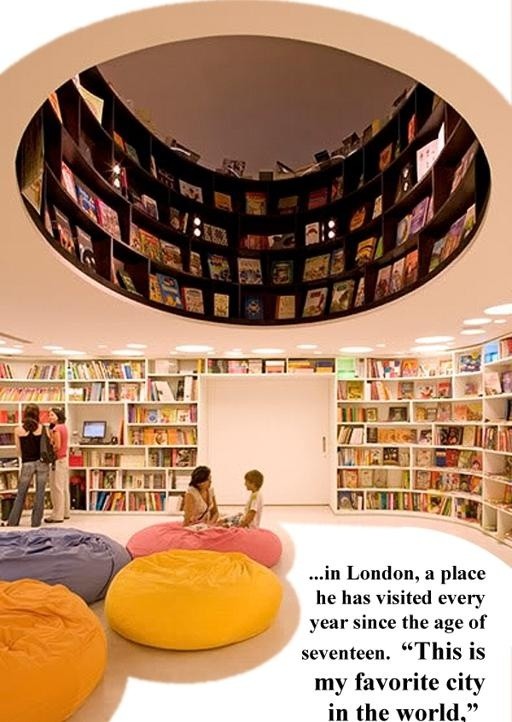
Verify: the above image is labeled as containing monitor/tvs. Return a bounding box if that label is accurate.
[83,420,107,442]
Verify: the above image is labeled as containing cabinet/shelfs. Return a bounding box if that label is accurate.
[11,64,495,331]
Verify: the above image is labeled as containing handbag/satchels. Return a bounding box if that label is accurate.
[40,425,57,470]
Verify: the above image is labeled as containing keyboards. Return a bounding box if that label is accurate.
[80,442,109,445]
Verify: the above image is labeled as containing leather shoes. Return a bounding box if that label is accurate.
[44,517,63,523]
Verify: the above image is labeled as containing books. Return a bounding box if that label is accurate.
[19,71,488,324]
[0,336,512,543]
